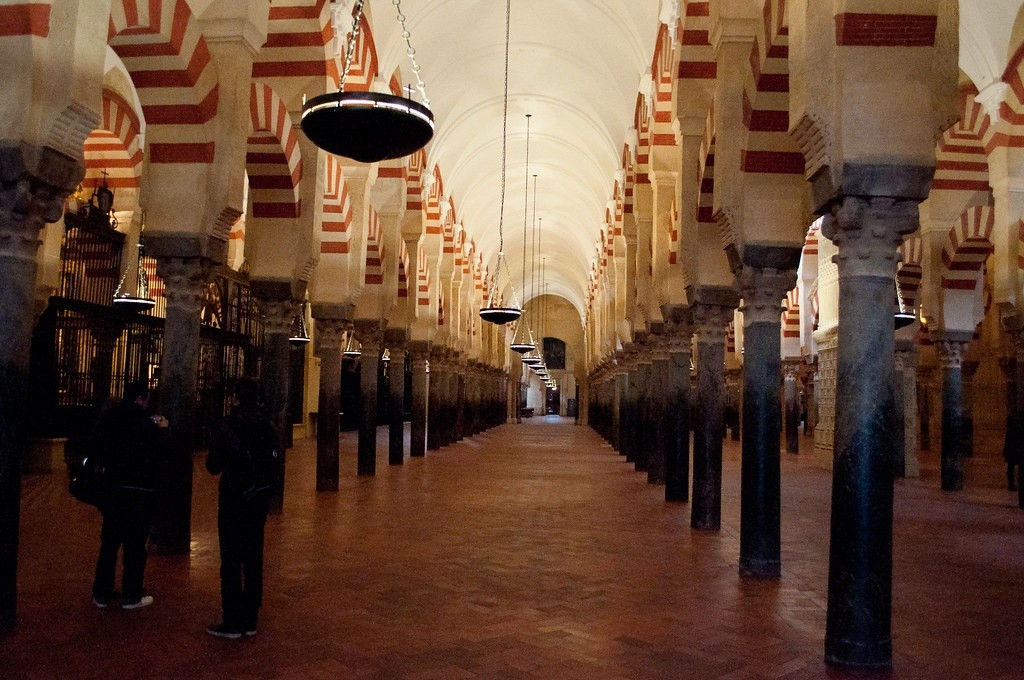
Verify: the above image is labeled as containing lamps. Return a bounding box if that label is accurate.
[510,114,536,353]
[479,0,522,325]
[522,174,558,389]
[110,238,156,313]
[289,302,311,348]
[894,274,917,329]
[344,328,362,359]
[301,0,434,163]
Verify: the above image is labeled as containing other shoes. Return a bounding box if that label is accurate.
[92,597,107,608]
[123,596,153,609]
[244,625,257,635]
[206,623,242,639]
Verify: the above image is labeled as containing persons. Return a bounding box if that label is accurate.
[91,381,169,609]
[205,376,278,640]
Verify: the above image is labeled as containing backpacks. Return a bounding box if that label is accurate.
[64,408,136,508]
[225,413,284,503]
